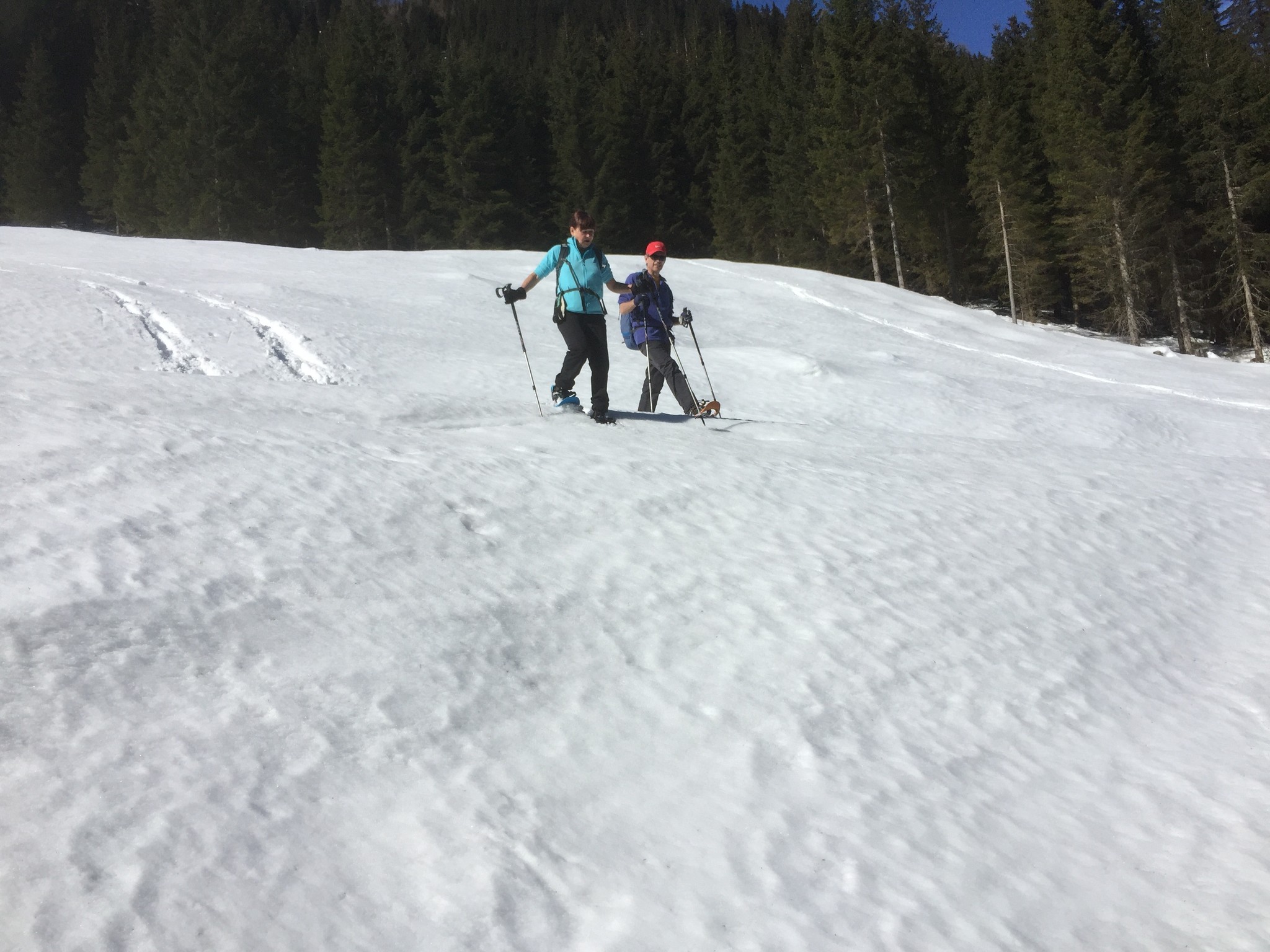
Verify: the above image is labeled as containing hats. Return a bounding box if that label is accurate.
[645,242,667,256]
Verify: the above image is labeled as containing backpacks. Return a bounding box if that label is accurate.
[620,311,639,350]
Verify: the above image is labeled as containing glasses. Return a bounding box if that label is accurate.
[649,254,666,261]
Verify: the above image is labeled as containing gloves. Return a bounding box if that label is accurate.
[679,310,693,325]
[634,293,650,309]
[632,272,653,295]
[504,288,526,304]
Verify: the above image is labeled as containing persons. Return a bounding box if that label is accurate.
[617,241,712,419]
[503,210,653,424]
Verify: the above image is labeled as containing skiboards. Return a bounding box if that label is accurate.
[556,397,616,424]
[696,401,721,417]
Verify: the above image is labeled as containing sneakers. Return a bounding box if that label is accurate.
[591,411,616,423]
[688,406,711,417]
[551,385,577,404]
